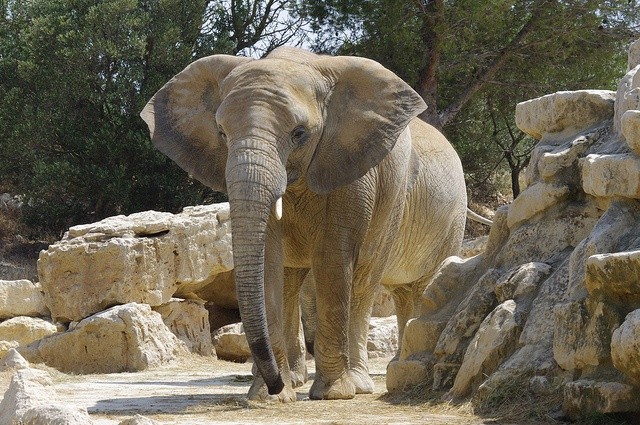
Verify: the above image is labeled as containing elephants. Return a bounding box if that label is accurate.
[137,42,469,406]
